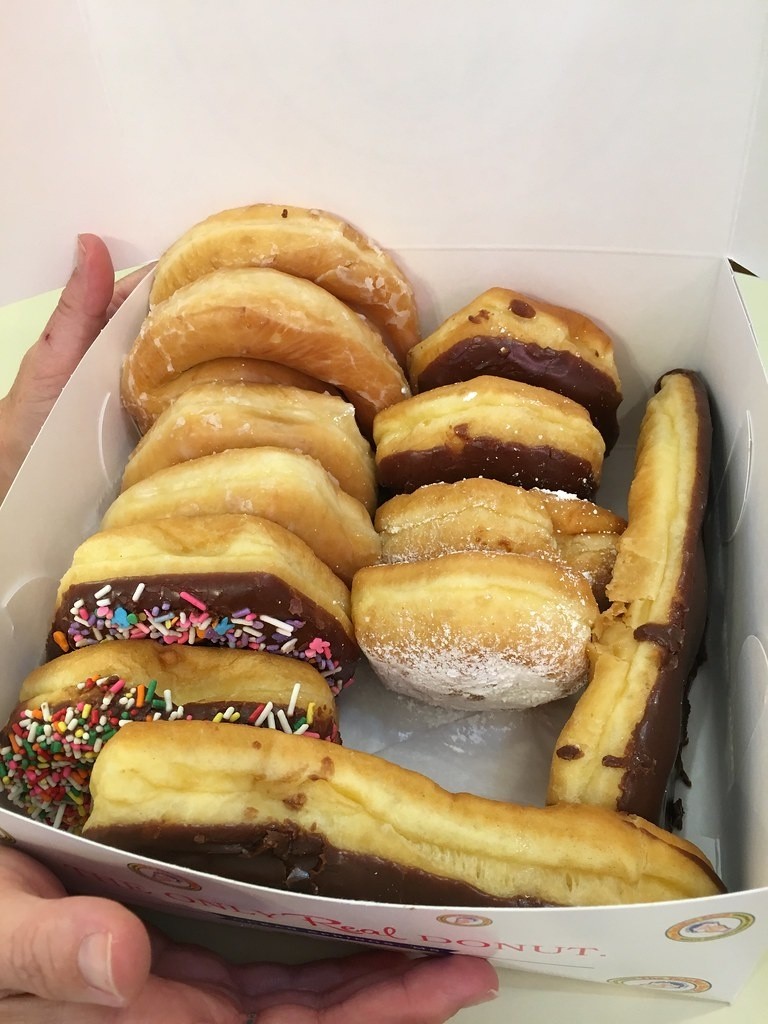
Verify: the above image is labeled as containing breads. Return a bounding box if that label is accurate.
[0,204,731,906]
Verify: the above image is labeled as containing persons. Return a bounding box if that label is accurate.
[0,233,501,1024]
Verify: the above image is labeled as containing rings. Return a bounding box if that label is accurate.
[245,1011,257,1024]
